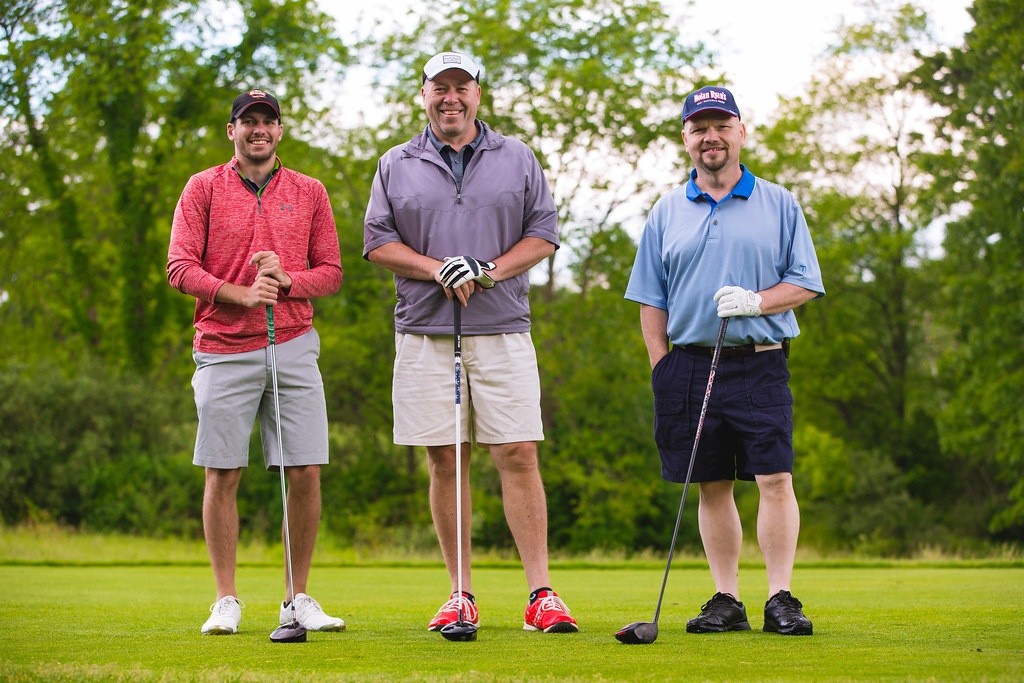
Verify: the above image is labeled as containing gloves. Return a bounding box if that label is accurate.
[714,286,761,319]
[439,256,496,289]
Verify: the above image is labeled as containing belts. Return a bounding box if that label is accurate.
[673,345,755,356]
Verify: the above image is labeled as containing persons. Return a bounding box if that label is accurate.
[164,90,347,635]
[362,51,581,634]
[623,86,826,637]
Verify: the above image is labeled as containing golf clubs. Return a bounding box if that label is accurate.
[613,315,730,645]
[263,274,307,643]
[439,288,478,642]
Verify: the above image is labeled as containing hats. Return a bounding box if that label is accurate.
[681,85,742,125]
[422,51,480,86]
[230,89,280,125]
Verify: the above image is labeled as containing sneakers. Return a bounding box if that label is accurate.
[426,591,478,632]
[686,593,751,634]
[279,594,347,632]
[523,591,578,634]
[763,589,813,635]
[200,595,244,634]
